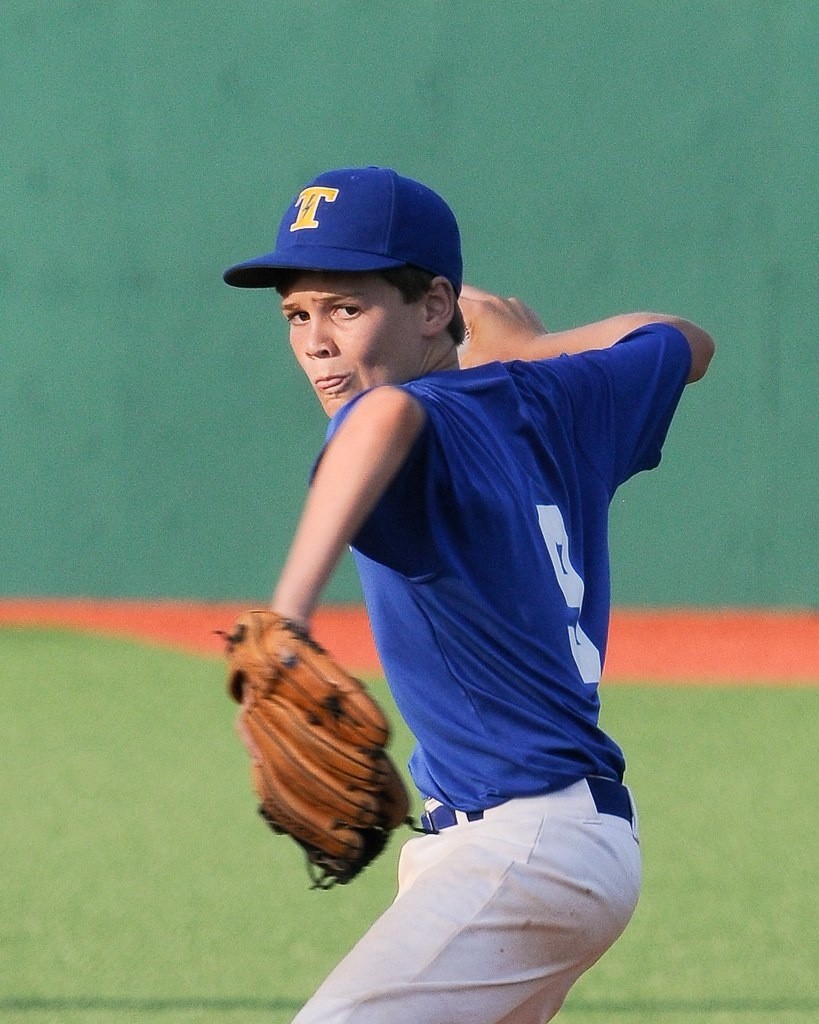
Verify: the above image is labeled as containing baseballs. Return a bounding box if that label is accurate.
[457,326,471,355]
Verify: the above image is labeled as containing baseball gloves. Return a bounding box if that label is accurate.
[208,608,413,893]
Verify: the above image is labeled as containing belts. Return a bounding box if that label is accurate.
[416,777,635,830]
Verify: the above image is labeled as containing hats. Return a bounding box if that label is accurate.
[223,165,462,297]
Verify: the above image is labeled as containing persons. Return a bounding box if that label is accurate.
[221,166,716,1024]
